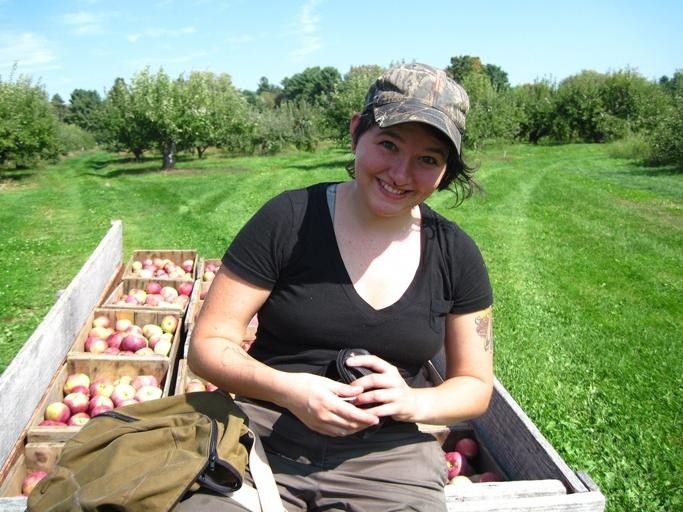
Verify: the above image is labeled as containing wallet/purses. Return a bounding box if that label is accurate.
[336,348,374,384]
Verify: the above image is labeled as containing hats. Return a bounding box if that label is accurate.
[364,63,469,156]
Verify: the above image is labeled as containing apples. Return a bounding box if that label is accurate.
[36,258,258,428]
[443,438,497,485]
[22,470,48,496]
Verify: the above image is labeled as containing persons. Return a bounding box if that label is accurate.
[166,63,495,512]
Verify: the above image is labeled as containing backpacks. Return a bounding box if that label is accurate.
[27,389,288,512]
